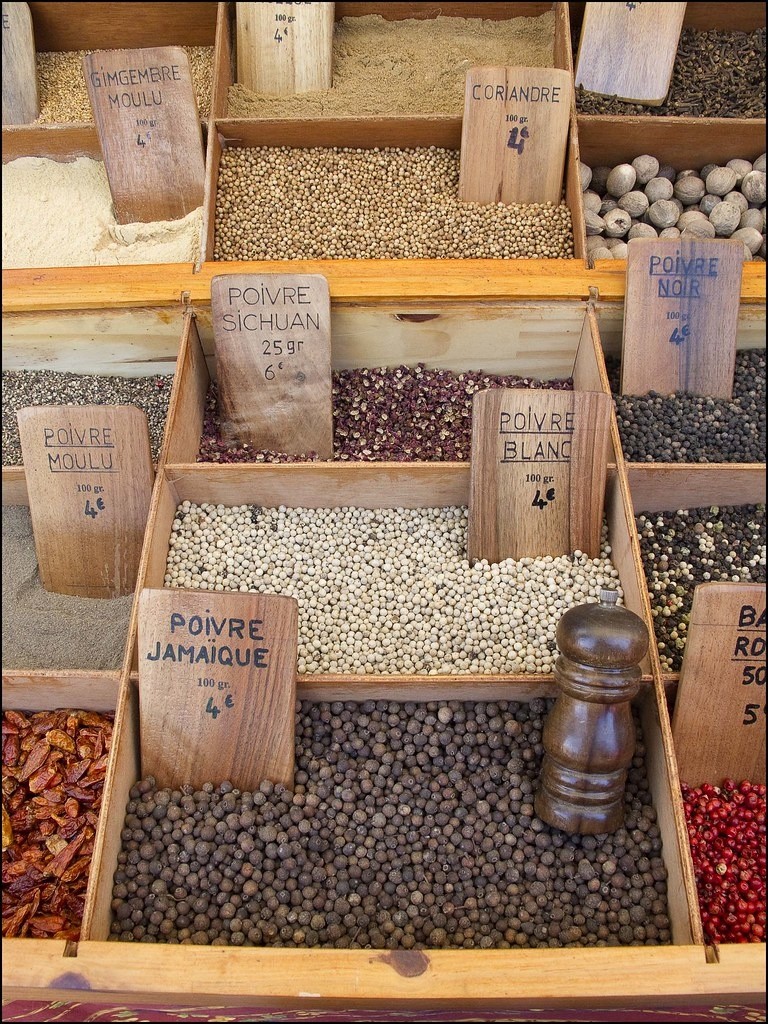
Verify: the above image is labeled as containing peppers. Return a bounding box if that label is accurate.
[2,709,115,940]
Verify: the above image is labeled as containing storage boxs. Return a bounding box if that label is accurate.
[1,2,765,994]
